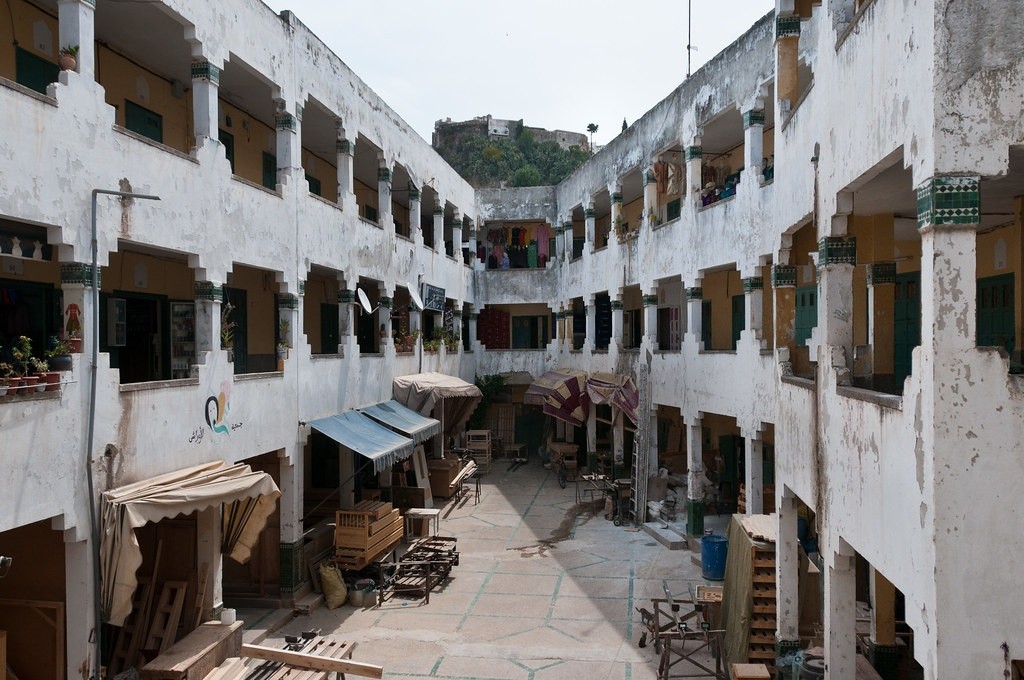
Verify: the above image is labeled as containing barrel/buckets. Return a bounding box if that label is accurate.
[701,535,728,581]
[799,657,824,680]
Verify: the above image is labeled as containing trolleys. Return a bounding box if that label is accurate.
[613,479,629,527]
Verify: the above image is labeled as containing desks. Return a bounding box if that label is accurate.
[403,507,439,543]
[505,441,530,459]
[138,619,244,680]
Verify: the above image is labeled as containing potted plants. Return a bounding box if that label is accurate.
[0,318,83,397]
[390,323,461,353]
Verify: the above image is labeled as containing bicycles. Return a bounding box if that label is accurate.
[554,451,575,488]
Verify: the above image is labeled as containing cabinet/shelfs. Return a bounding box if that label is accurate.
[465,428,492,477]
